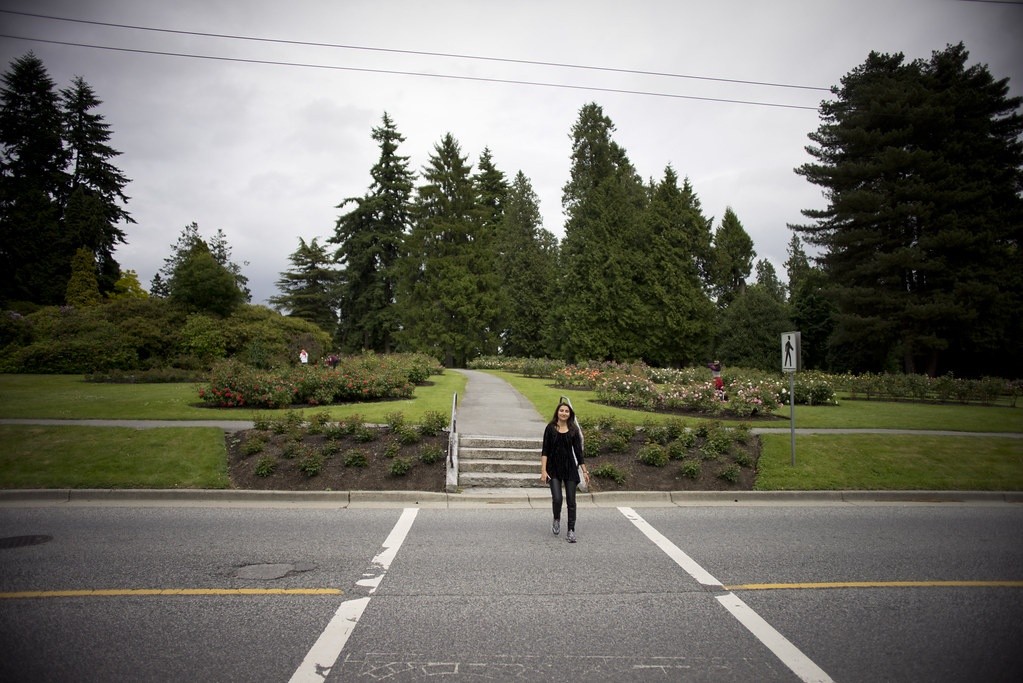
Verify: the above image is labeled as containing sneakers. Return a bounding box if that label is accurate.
[566,530,577,543]
[551,517,561,535]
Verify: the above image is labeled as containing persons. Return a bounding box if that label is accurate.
[299,348,338,369]
[540,402,589,543]
[708,360,724,392]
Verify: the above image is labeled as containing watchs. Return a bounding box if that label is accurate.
[583,470,589,474]
